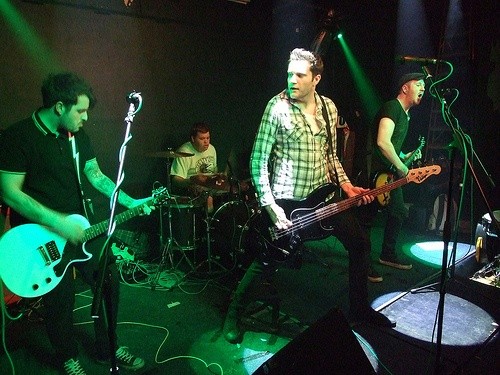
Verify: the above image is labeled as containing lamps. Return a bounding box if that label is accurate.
[330,19,343,40]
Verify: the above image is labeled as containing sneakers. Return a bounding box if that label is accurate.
[63,358,87,375]
[96,346,145,370]
[367,265,384,283]
[378,254,413,269]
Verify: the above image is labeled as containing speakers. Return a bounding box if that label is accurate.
[345,161,383,227]
[250,309,394,375]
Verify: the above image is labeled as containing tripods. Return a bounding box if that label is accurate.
[151,192,198,290]
[186,201,235,279]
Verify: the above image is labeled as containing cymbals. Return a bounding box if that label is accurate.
[142,151,195,159]
[190,173,250,194]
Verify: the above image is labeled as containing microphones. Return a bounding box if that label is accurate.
[126,90,142,103]
[393,54,443,65]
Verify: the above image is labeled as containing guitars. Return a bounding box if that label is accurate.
[370,136,426,209]
[0,185,172,298]
[251,162,443,262]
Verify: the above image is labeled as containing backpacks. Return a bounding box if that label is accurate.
[425,192,458,232]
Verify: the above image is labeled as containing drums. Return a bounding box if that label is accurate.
[208,200,282,275]
[482,211,500,264]
[157,200,208,252]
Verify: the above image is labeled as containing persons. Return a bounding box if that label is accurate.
[0,72,156,375]
[223,48,396,343]
[367,73,425,281]
[170,124,219,207]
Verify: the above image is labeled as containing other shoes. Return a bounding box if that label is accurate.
[348,305,396,327]
[222,302,243,343]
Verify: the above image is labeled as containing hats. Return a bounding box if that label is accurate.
[398,73,425,88]
